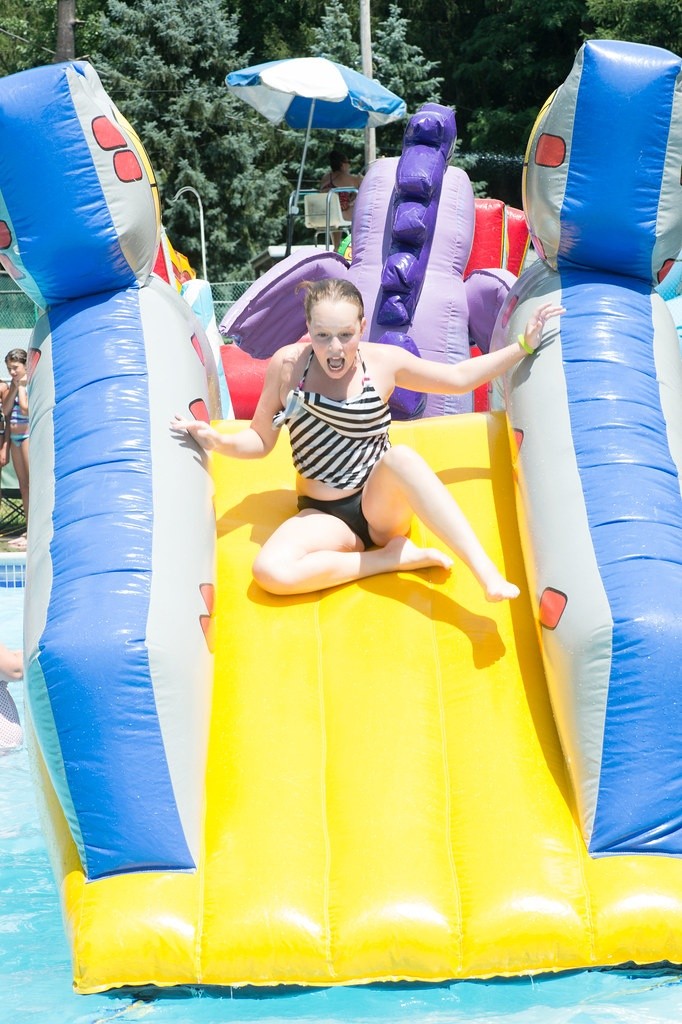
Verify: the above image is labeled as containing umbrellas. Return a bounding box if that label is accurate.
[224,55,407,206]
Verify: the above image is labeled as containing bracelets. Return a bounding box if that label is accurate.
[518,334,534,354]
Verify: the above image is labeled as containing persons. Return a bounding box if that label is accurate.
[169,279,567,603]
[0,644,24,747]
[320,152,364,251]
[0,381,11,477]
[3,348,30,547]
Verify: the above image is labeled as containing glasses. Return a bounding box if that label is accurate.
[343,159,352,165]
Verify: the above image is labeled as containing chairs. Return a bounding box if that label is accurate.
[303,192,352,252]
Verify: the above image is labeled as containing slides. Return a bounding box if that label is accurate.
[29,275,677,988]
